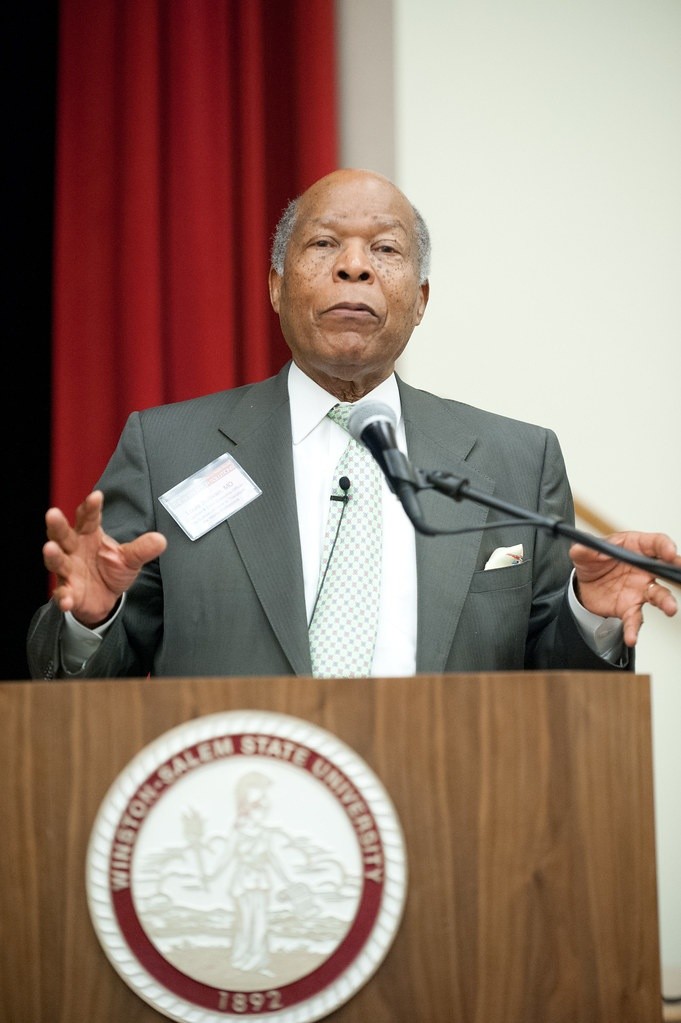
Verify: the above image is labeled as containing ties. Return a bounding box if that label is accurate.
[309,402,386,680]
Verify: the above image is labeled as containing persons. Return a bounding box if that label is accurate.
[30,168,680,684]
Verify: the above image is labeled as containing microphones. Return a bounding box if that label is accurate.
[331,477,350,502]
[347,399,425,524]
